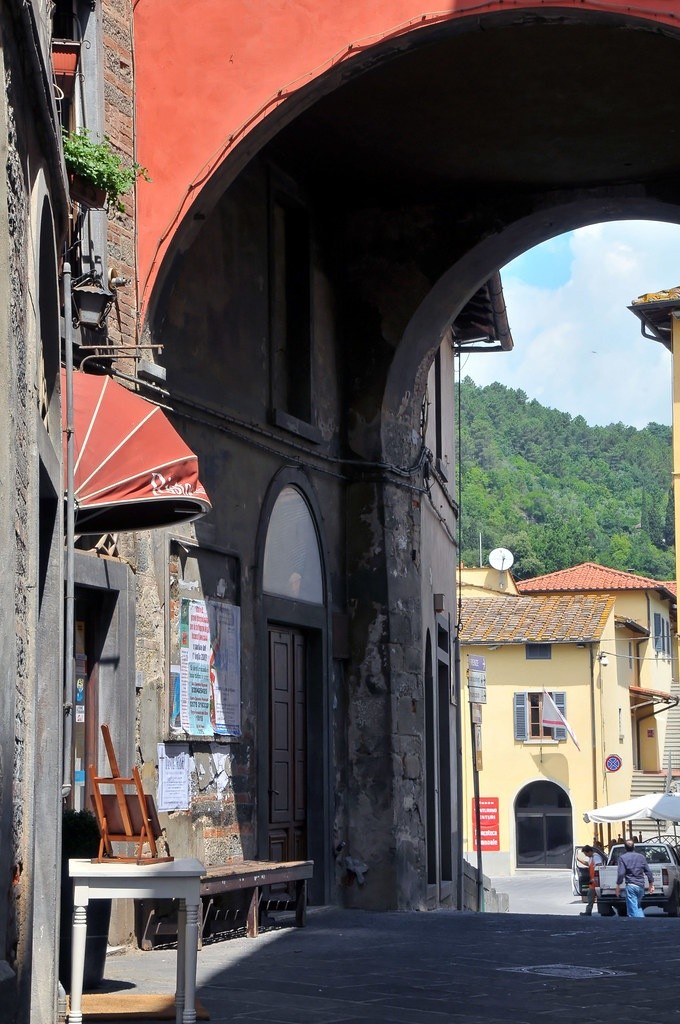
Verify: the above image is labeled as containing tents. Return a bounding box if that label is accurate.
[582,793,680,849]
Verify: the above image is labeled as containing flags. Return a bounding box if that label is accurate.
[540,691,580,749]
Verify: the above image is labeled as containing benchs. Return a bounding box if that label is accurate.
[140,860,313,951]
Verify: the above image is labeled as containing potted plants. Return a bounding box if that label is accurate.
[59,124,153,213]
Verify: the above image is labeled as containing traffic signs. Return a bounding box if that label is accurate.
[468,669,487,689]
[467,686,486,704]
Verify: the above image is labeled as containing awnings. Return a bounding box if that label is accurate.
[55,368,211,537]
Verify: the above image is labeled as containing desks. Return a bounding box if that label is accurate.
[67,855,208,1024]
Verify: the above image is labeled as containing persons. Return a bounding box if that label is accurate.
[576,844,605,916]
[615,840,654,918]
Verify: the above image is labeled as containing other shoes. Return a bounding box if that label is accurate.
[580,912,592,916]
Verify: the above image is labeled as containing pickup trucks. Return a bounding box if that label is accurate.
[571,842,680,917]
